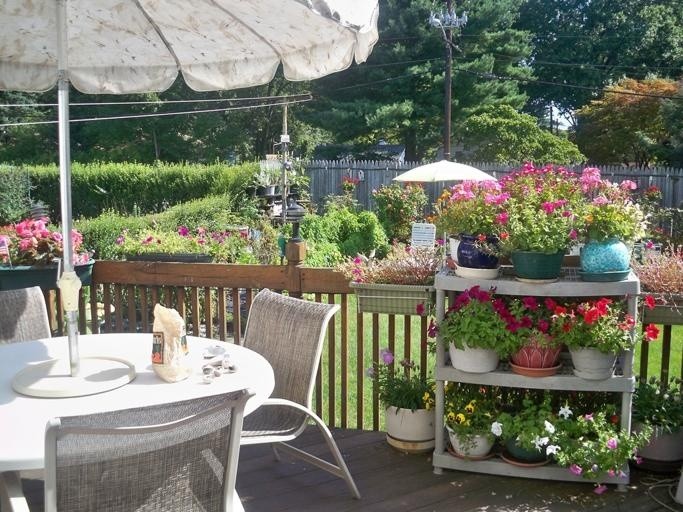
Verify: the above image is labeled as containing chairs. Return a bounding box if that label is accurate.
[411,223,436,252]
[1,284,52,347]
[240,288,361,499]
[0,388,256,512]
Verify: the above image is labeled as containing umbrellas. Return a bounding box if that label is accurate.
[0,0,379,377]
[392,159,498,269]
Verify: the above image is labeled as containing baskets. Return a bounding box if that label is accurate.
[348,280,435,316]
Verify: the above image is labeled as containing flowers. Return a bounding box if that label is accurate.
[630,375,683,439]
[630,238,683,314]
[554,298,659,353]
[422,382,495,465]
[487,393,572,457]
[510,295,567,348]
[428,284,529,359]
[365,348,436,415]
[495,160,577,256]
[1,217,97,267]
[333,247,444,286]
[556,404,654,494]
[578,166,651,242]
[117,225,230,253]
[439,179,496,255]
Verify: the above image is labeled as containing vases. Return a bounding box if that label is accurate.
[444,424,496,456]
[511,249,568,279]
[508,335,562,368]
[569,347,619,370]
[124,252,216,263]
[448,337,499,373]
[454,233,502,269]
[633,421,683,461]
[384,402,436,454]
[500,437,552,462]
[577,237,632,272]
[0,257,96,292]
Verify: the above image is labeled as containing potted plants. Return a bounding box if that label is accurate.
[246,160,283,196]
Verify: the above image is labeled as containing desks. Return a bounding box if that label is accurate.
[0,333,275,472]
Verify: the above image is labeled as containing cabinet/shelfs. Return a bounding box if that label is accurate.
[435,255,641,492]
